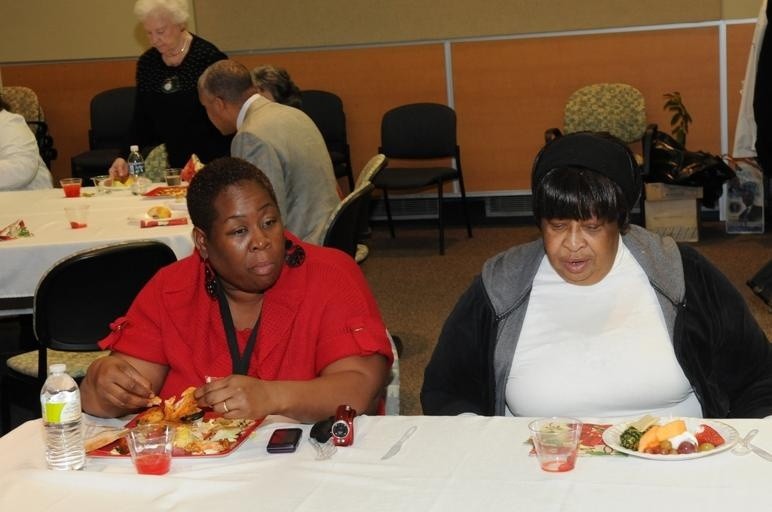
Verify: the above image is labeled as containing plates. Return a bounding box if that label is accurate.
[85,407,267,459]
[599,415,740,463]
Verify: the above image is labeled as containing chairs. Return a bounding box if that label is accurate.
[0,87,50,172]
[7,239,179,431]
[544,82,658,227]
[371,103,473,256]
[297,91,354,193]
[71,86,135,187]
[354,154,388,264]
[318,181,375,260]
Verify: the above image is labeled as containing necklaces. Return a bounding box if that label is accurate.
[152,35,192,59]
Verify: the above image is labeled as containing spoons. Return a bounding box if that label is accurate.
[734,427,763,456]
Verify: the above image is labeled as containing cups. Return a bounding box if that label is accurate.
[163,168,183,188]
[92,175,114,198]
[58,177,83,199]
[124,422,175,476]
[64,203,90,228]
[525,416,584,474]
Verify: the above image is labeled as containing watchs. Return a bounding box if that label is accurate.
[330,406,355,447]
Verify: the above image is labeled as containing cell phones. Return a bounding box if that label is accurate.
[267,427,302,452]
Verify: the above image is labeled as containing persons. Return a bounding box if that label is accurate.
[731,0,772,311]
[79,156,395,422]
[107,0,245,186]
[738,190,762,222]
[251,65,345,206]
[197,58,345,250]
[420,131,772,417]
[0,98,55,193]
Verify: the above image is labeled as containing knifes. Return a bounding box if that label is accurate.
[729,422,768,462]
[380,424,418,462]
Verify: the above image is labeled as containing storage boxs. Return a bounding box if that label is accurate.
[645,183,703,201]
[645,200,702,242]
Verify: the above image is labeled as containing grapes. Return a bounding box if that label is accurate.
[698,443,715,451]
[677,441,695,454]
[660,440,672,450]
[669,449,679,454]
[643,448,654,454]
[660,448,669,455]
[653,447,661,454]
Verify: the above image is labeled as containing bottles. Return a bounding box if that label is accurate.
[40,364,85,472]
[128,146,145,194]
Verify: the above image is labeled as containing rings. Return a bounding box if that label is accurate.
[222,401,230,414]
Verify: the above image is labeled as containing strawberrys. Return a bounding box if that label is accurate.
[696,424,725,446]
